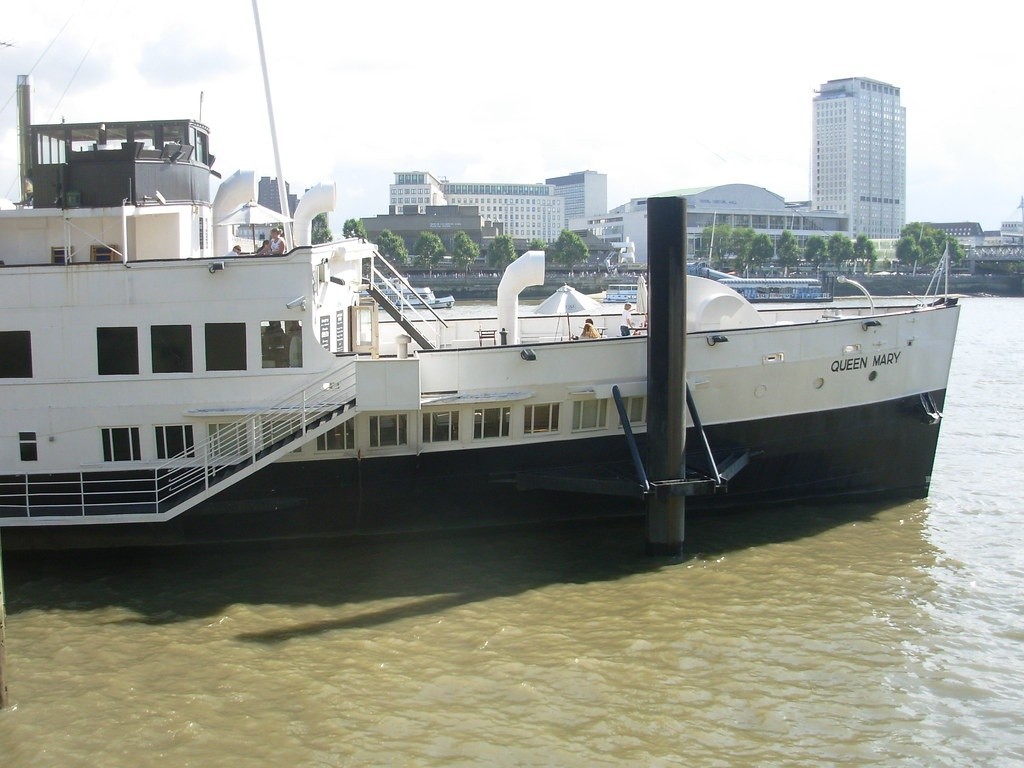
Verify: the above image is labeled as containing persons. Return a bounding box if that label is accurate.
[225,227,286,255]
[579,303,647,341]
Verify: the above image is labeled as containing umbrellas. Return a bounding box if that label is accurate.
[210,199,295,252]
[532,282,603,339]
[636,274,647,327]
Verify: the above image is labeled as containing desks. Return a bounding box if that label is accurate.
[474,329,498,347]
[628,326,647,335]
[579,326,608,338]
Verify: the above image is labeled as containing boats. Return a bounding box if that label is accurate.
[0,71,963,545]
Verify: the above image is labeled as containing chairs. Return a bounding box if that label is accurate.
[13,193,33,209]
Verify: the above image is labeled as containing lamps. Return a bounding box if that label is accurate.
[210,170,222,179]
[170,151,185,163]
